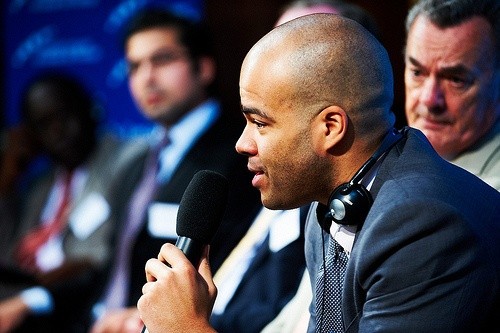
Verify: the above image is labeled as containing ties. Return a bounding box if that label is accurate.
[12,171,73,277]
[103,135,171,313]
[315,233,349,333]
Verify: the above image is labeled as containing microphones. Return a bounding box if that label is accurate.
[140,169,232,333]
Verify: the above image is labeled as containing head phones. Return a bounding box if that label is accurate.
[316,123,407,233]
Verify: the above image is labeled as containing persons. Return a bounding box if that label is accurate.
[136,13,500,333]
[0,0,364,333]
[258,0,500,333]
